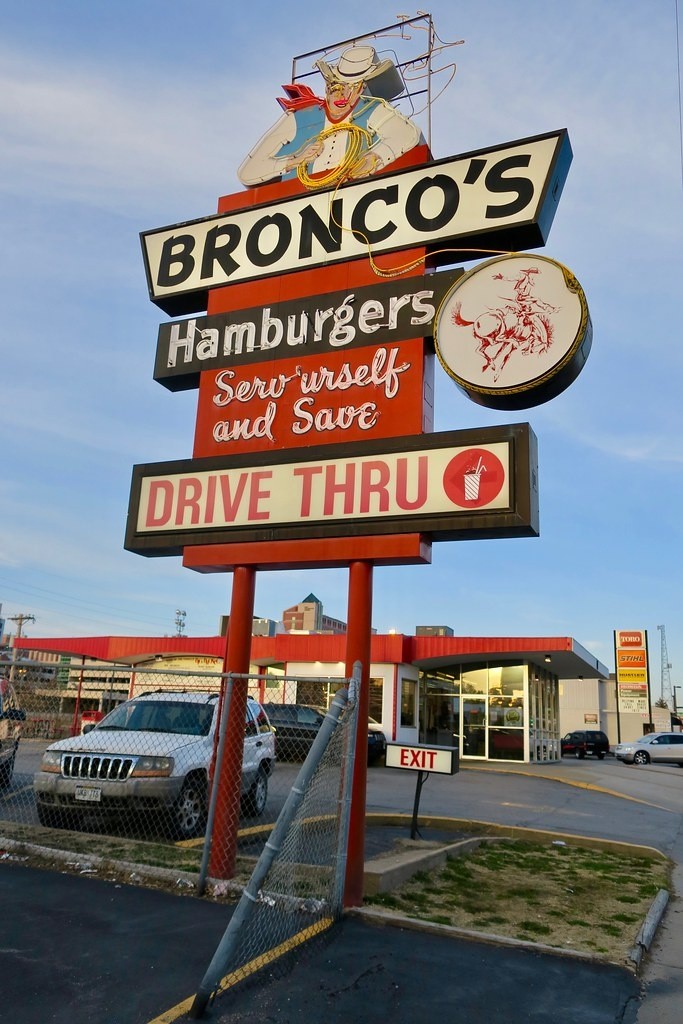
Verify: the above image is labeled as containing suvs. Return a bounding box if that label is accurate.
[0,675,26,788]
[466,728,523,760]
[561,730,611,760]
[615,732,683,765]
[32,690,277,842]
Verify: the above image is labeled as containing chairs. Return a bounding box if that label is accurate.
[173,707,204,735]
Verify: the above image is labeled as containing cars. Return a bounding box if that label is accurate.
[260,703,388,764]
[529,730,558,760]
